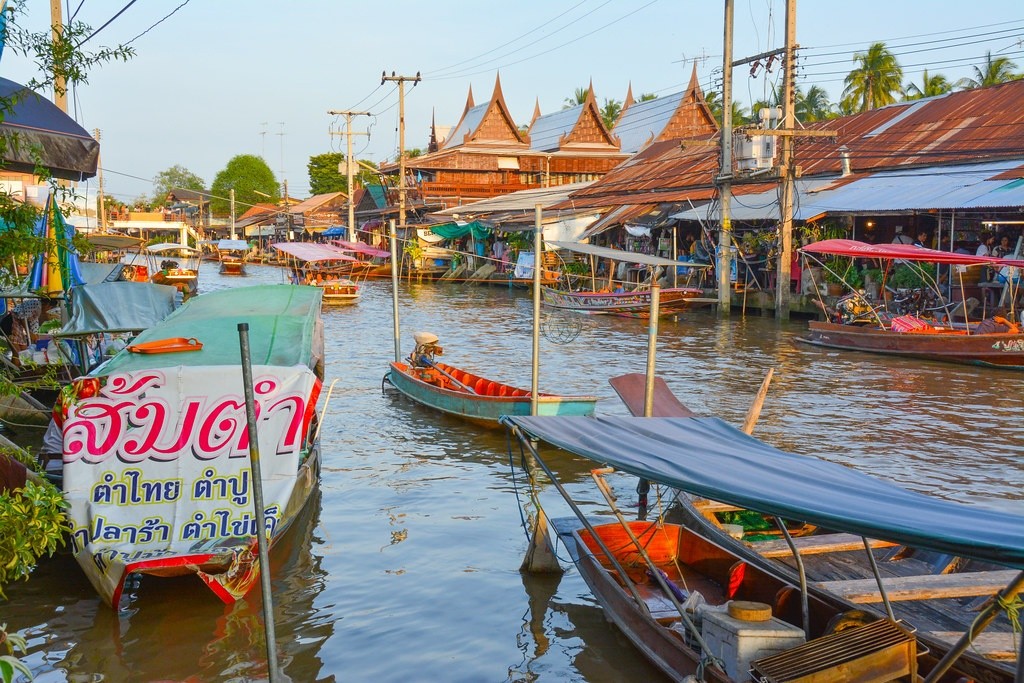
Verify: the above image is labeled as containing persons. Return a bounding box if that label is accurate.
[245,234,394,262]
[106,194,182,223]
[459,235,507,270]
[861,223,1023,309]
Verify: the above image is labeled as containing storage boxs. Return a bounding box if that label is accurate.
[699,610,807,683]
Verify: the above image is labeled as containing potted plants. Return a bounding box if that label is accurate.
[883,277,896,301]
[862,268,883,300]
[823,260,850,298]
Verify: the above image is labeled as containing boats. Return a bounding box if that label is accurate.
[1,190,178,554]
[607,373,1024,674]
[534,239,720,324]
[792,239,1024,373]
[146,242,204,294]
[216,239,253,276]
[40,283,330,613]
[496,411,1024,683]
[328,237,398,281]
[273,242,380,307]
[381,331,597,436]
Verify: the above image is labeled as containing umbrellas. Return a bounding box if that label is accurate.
[26,189,88,298]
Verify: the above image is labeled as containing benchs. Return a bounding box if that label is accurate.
[978,282,1024,308]
